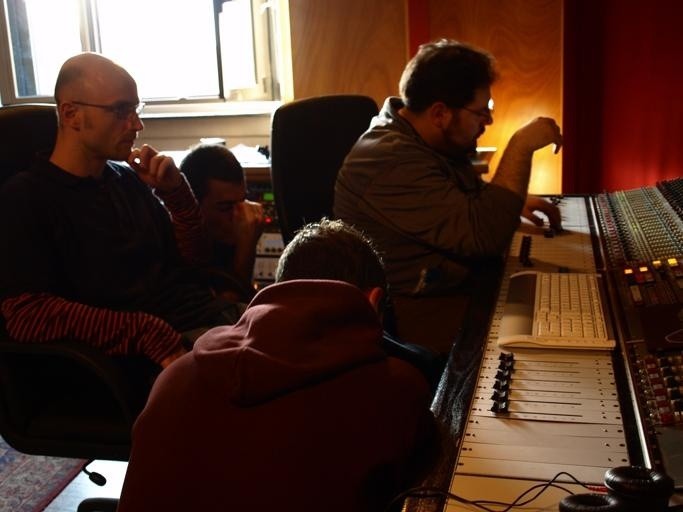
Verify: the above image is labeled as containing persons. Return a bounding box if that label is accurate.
[331,37,565,340]
[173,145,265,276]
[0,53,225,457]
[114,214,443,511]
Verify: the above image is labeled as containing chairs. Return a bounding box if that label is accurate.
[1,98,58,172]
[269,94,379,249]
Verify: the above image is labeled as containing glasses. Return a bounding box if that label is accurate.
[461,104,491,123]
[70,100,146,120]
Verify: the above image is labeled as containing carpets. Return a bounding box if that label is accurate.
[0,436,95,511]
